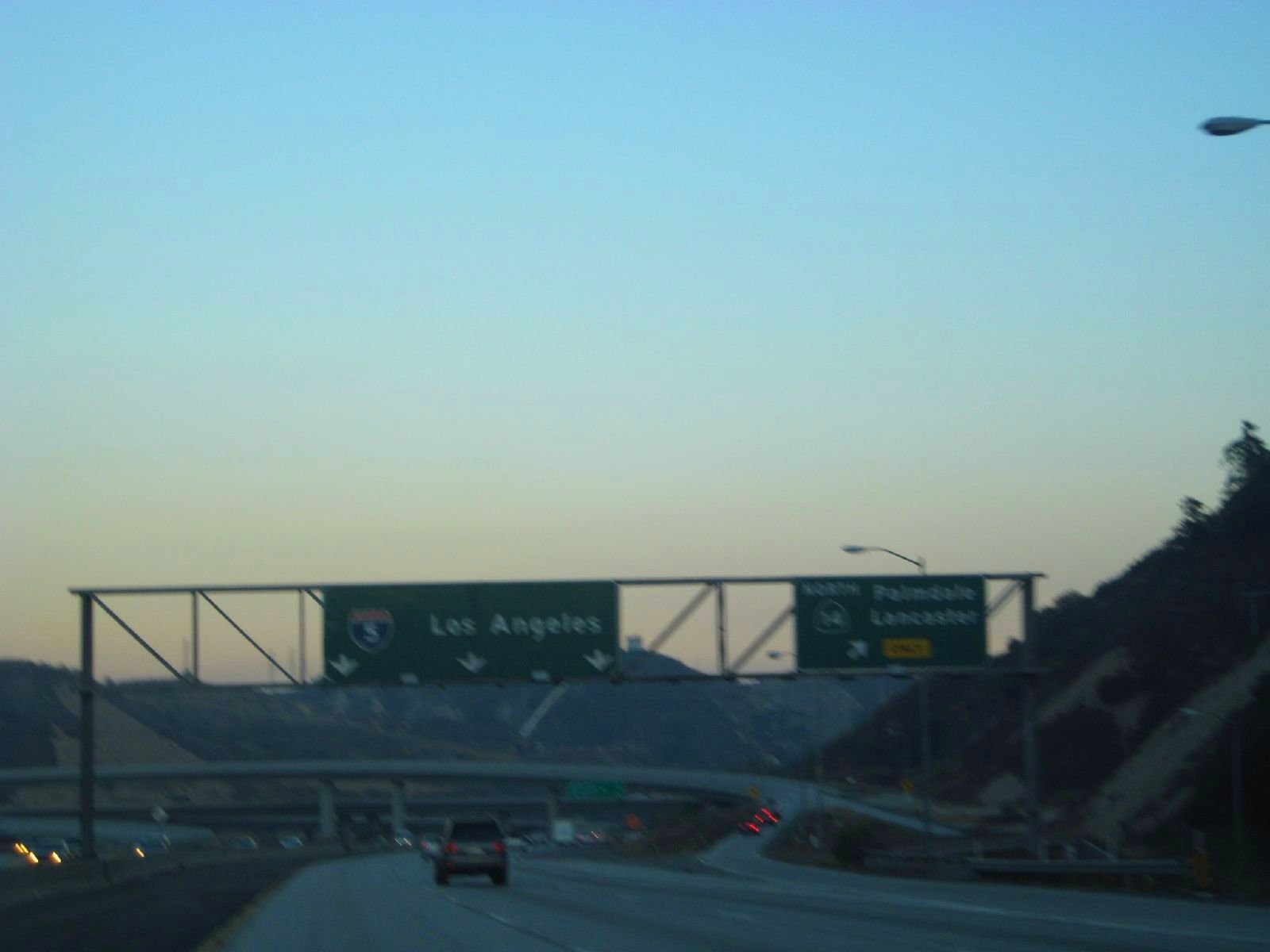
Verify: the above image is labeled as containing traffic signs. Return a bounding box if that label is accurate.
[323,580,620,687]
[793,576,986,673]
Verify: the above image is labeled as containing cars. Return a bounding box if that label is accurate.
[417,831,445,861]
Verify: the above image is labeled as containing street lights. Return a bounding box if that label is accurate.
[842,543,930,797]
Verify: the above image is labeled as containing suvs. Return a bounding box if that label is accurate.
[433,817,510,886]
